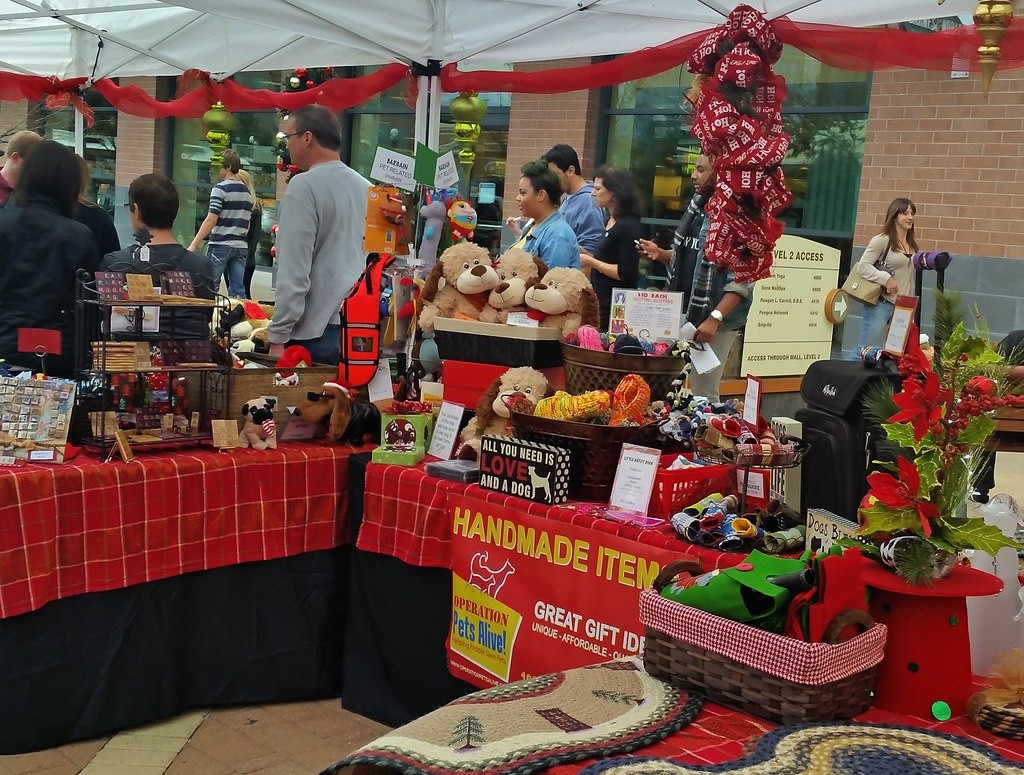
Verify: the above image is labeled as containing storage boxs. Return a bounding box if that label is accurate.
[434,316,565,412]
[372,413,433,466]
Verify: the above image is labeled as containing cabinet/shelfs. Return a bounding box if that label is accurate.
[75,223,231,463]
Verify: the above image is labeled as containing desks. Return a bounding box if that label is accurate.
[1,439,1024,775]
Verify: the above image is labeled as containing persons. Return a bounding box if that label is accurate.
[0,105,379,380]
[504,144,747,403]
[845,196,921,361]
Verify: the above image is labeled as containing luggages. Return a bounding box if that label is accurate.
[795,250,952,526]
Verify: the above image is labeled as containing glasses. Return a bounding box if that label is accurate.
[686,339,706,352]
[124,203,130,211]
[282,130,304,143]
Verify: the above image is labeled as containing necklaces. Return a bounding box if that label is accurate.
[897,237,912,268]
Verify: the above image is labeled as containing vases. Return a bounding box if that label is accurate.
[858,563,1005,720]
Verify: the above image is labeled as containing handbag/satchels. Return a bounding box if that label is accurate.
[842,236,894,306]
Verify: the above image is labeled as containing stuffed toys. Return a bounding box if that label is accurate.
[240,192,600,461]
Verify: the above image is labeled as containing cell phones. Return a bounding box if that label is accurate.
[634,240,649,255]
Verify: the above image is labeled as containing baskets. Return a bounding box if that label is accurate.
[638,560,887,726]
[688,427,804,469]
[500,394,671,503]
[558,339,686,403]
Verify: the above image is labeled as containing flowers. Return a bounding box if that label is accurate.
[857,284,1024,588]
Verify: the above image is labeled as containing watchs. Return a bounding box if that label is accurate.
[711,310,724,323]
